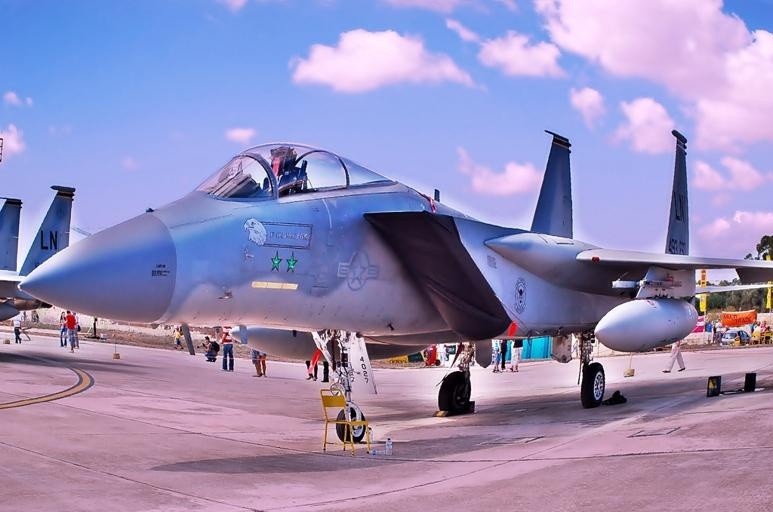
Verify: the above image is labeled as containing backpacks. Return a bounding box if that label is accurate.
[209,341,220,352]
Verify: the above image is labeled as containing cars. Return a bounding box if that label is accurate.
[721,329,751,345]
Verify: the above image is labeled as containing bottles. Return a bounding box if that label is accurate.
[344,404,351,422]
[366,449,386,455]
[368,427,373,443]
[385,438,392,456]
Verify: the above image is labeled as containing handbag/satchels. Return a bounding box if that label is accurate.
[77,324,81,332]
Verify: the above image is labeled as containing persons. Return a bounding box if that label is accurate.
[706,319,723,347]
[305,361,329,382]
[252,349,268,377]
[31,310,39,323]
[219,326,234,371]
[751,320,770,344]
[59,311,79,353]
[202,336,217,362]
[173,328,184,349]
[492,339,523,373]
[662,340,685,373]
[11,312,22,344]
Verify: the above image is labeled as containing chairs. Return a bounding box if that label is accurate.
[318,388,370,457]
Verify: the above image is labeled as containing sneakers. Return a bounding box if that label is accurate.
[61,345,79,353]
[258,373,267,378]
[306,375,329,381]
[492,367,518,373]
[220,368,233,372]
[206,358,216,361]
[173,346,184,349]
[662,367,685,373]
[15,338,22,344]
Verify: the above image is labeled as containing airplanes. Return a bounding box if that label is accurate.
[16,131,773,443]
[1,184,76,317]
